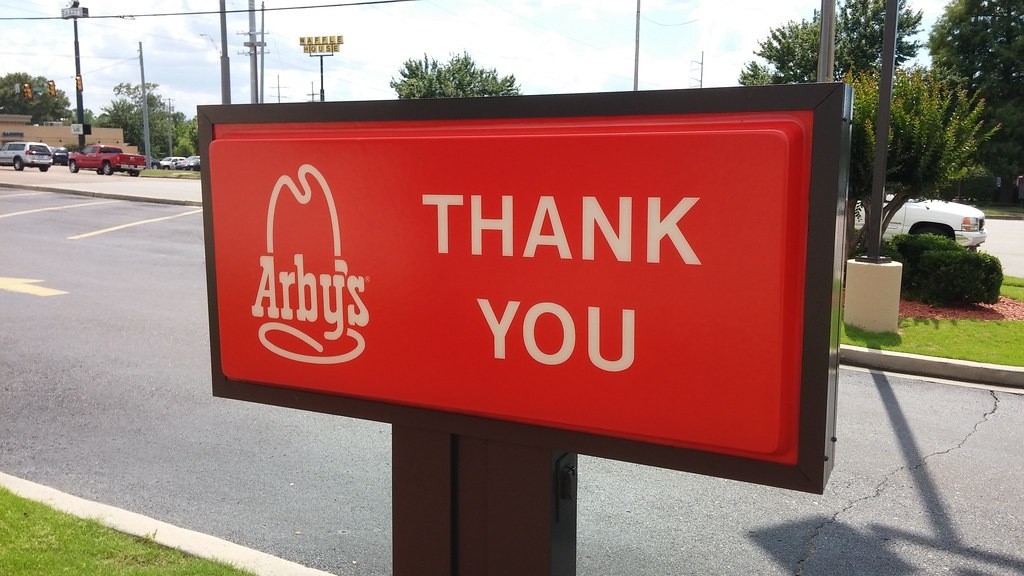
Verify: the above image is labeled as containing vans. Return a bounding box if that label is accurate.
[159,157,186,169]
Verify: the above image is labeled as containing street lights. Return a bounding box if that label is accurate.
[200,33,230,104]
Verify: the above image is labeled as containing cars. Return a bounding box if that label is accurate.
[50,147,69,165]
[142,156,162,170]
[176,156,200,170]
[850,195,985,252]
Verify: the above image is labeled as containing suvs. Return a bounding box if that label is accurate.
[0,142,54,172]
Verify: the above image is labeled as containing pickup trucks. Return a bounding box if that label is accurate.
[69,145,147,177]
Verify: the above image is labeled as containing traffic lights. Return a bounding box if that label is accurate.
[23,84,32,99]
[75,76,83,91]
[48,80,56,96]
